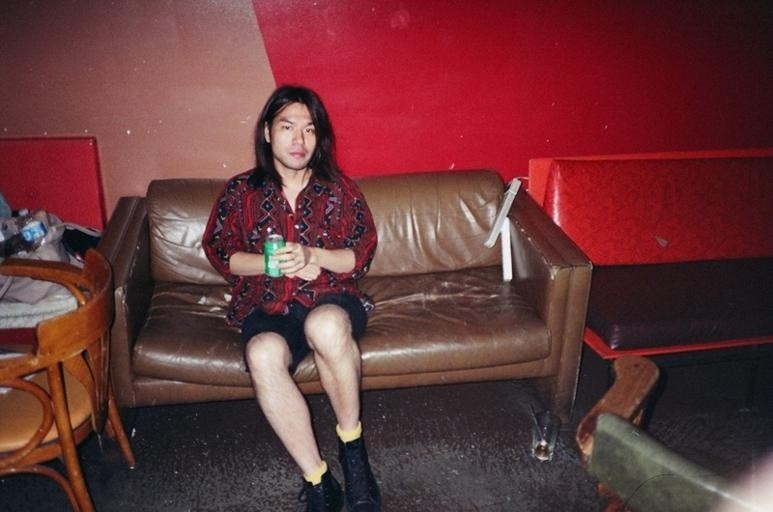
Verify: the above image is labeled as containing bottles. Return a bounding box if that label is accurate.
[16,206,49,251]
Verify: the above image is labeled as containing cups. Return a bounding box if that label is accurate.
[528,408,560,462]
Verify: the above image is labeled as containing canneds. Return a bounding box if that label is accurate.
[264,233,285,279]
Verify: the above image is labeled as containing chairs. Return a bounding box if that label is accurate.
[574,355,773,512]
[0,247,137,512]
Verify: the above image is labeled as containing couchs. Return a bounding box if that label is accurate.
[92,170,595,423]
[0,136,109,353]
[545,154,773,455]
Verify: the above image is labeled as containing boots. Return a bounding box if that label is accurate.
[297,464,342,512]
[334,436,381,512]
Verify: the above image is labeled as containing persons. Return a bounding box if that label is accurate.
[200,85,381,512]
